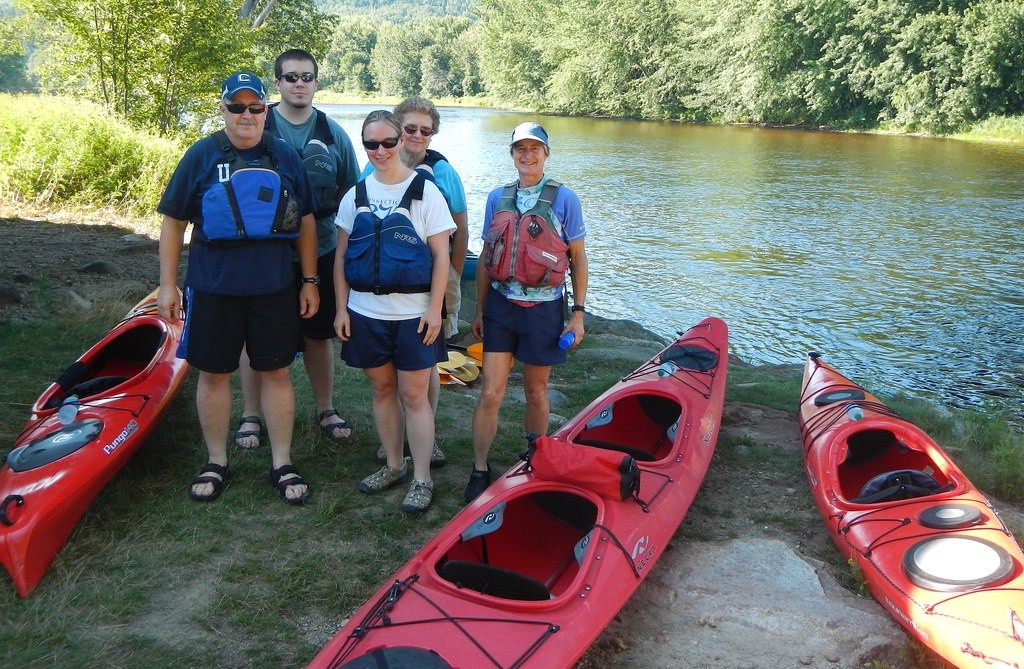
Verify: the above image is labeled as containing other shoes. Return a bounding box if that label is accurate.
[377,445,387,461]
[465,461,491,502]
[431,439,445,466]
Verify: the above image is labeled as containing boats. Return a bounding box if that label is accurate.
[0,284,192,600]
[305,316,730,669]
[794,352,1023,669]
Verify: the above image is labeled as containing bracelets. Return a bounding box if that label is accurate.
[572,305,585,313]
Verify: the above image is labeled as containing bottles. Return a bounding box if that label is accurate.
[58,394,79,425]
[657,360,677,378]
[846,402,863,421]
[559,332,575,349]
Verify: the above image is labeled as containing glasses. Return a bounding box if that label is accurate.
[362,135,400,150]
[277,71,316,83]
[402,123,435,136]
[222,102,266,114]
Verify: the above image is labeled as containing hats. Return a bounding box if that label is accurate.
[221,71,266,100]
[508,122,549,146]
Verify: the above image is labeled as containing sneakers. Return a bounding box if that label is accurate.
[357,456,411,494]
[400,474,433,512]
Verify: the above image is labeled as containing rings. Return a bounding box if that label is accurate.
[581,338,584,339]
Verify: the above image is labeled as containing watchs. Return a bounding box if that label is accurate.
[302,275,319,286]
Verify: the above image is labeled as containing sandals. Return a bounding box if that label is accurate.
[189,463,230,502]
[270,464,310,505]
[314,405,352,440]
[234,416,262,449]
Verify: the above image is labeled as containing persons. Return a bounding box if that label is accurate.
[235,49,362,450]
[157,71,319,503]
[335,98,468,510]
[464,122,588,503]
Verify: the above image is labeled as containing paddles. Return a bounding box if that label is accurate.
[435,341,515,388]
